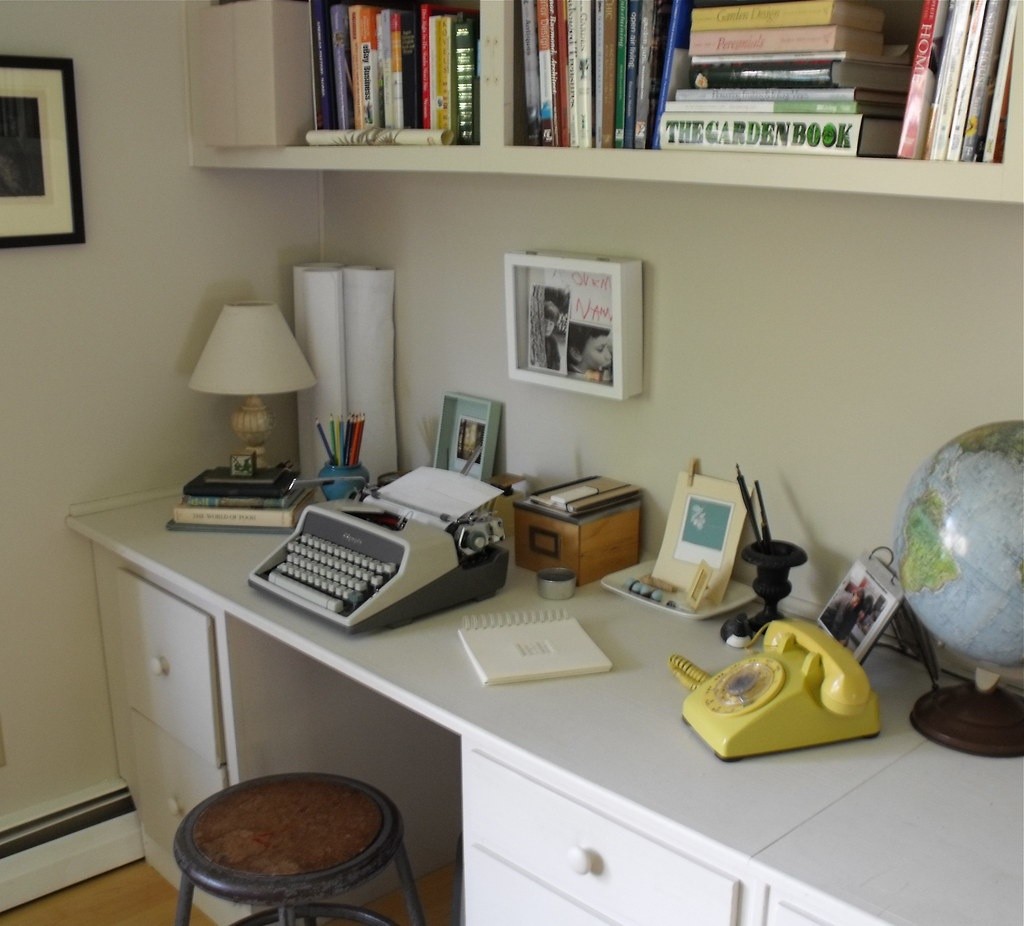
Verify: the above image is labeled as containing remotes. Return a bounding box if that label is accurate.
[551,485,599,503]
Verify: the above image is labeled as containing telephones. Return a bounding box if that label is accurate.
[667,619,880,765]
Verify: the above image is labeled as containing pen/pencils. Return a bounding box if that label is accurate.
[315,410,366,466]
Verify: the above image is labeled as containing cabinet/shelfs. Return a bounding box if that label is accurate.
[185,0,1024,202]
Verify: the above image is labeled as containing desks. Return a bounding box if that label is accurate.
[66,487,1024,926]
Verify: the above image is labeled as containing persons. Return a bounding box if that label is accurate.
[567,322,612,385]
[822,586,872,644]
[542,299,562,371]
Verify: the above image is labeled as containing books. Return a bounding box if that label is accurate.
[519,0,694,150]
[896,0,1019,164]
[529,472,643,514]
[174,468,316,529]
[457,606,614,684]
[309,0,480,145]
[658,0,913,158]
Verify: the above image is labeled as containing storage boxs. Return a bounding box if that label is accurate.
[436,391,503,483]
[512,499,640,585]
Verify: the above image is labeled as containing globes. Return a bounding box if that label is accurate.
[892,420,1024,759]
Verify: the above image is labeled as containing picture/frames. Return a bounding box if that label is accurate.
[0,54,86,247]
[504,250,644,401]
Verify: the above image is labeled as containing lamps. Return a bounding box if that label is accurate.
[191,300,321,473]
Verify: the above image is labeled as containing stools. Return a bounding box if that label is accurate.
[172,771,423,926]
